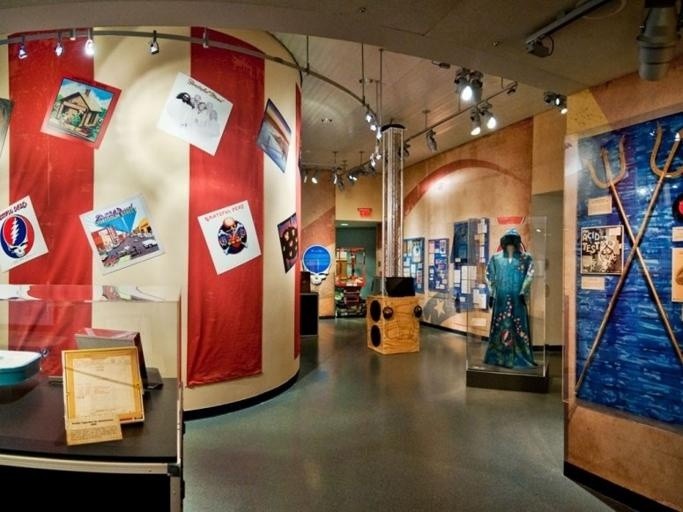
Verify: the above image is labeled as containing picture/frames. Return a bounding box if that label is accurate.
[671,248,683,303]
[580,225,624,276]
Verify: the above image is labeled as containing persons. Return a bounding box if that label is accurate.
[484,230,538,370]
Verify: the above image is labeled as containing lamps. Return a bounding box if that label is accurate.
[6,28,214,60]
[299,33,410,187]
[421,56,571,155]
